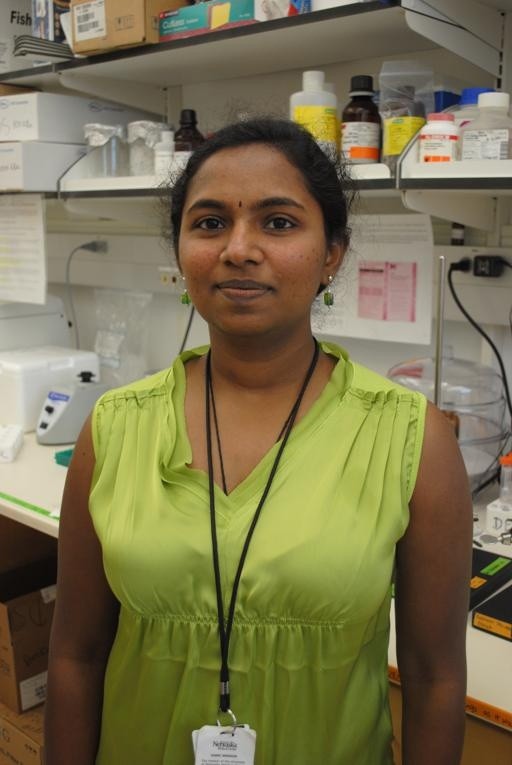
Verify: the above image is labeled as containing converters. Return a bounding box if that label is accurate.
[473,255,504,278]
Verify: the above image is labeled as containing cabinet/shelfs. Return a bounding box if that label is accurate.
[0,1,512,195]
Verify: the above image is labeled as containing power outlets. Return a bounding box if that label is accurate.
[439,245,511,284]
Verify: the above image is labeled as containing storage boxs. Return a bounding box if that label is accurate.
[68,0,185,57]
[0,583,58,716]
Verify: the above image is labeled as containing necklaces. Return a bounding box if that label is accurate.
[209,370,301,496]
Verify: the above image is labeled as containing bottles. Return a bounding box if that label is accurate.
[84,108,207,176]
[290,70,511,161]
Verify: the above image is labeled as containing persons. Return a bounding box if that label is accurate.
[43,116,474,765]
